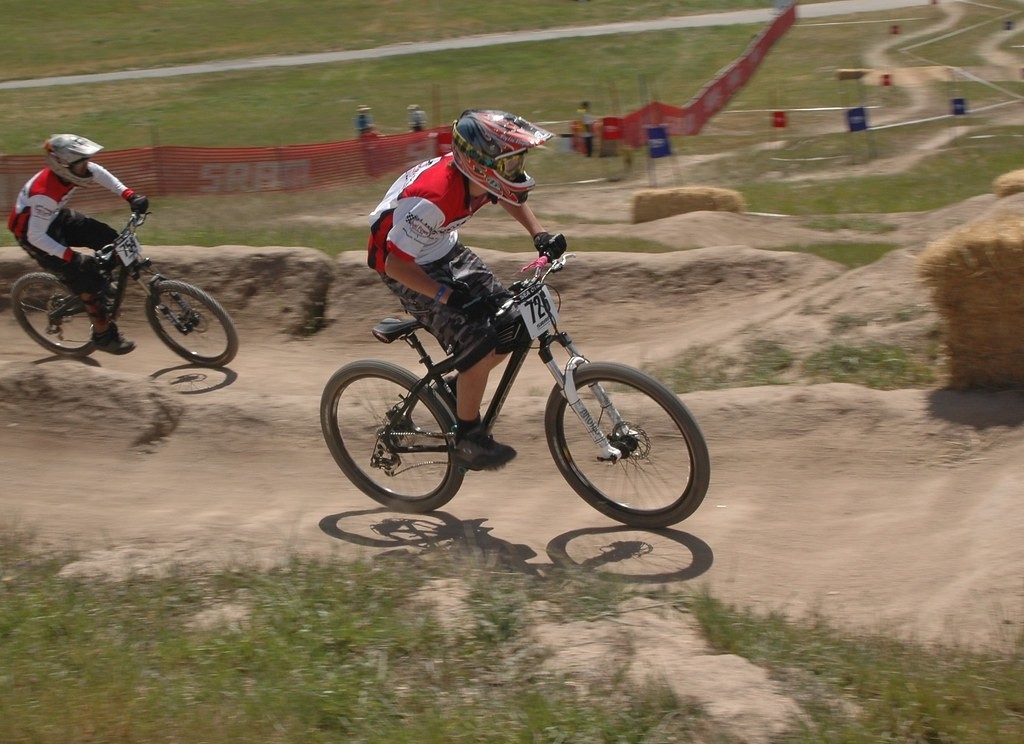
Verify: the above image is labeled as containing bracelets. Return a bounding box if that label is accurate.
[434,283,448,301]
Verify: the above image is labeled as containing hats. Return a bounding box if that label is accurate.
[408,104,419,109]
[356,104,371,112]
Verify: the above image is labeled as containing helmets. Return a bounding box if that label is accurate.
[45,133,103,188]
[448,109,557,206]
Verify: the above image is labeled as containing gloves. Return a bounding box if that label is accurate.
[73,251,98,273]
[533,232,567,259]
[126,192,149,214]
[447,289,502,324]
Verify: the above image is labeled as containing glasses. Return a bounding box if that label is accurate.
[496,152,526,177]
[68,160,88,175]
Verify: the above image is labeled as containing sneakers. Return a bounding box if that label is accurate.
[449,425,516,470]
[100,270,118,296]
[91,322,137,354]
[433,376,456,414]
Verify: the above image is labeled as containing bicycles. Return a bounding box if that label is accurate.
[9,203,241,370]
[320,248,711,529]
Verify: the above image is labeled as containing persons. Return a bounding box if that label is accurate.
[576,101,596,158]
[366,106,567,469]
[6,133,149,356]
